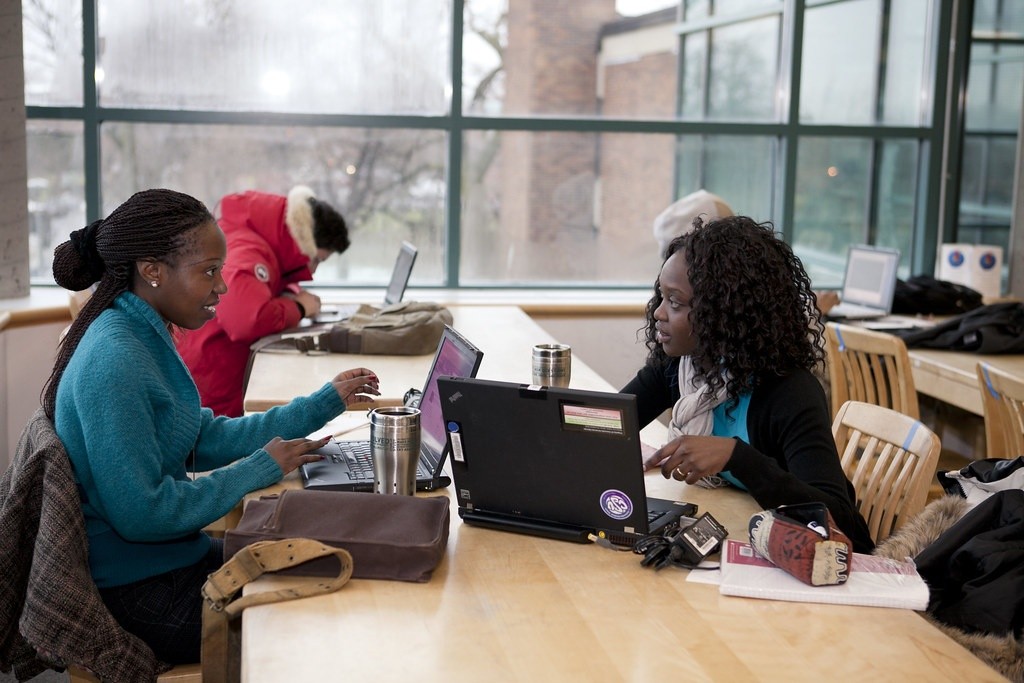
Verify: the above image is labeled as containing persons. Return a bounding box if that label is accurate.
[40,189,381,658]
[617,190,877,555]
[172,186,352,419]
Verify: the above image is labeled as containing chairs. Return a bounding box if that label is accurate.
[975,363,1024,462]
[0,405,204,683]
[831,396,941,547]
[824,321,923,419]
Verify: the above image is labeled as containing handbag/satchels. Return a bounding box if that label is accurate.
[892,274,986,316]
[894,300,1024,354]
[242,301,453,399]
[201,490,451,683]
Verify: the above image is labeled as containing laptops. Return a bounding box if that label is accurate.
[819,246,902,320]
[298,322,484,490]
[310,242,419,322]
[436,375,699,548]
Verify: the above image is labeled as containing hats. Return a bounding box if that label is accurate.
[654,188,732,262]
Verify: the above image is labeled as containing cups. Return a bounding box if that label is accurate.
[532,344,572,388]
[367,407,422,496]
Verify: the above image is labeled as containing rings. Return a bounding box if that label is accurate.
[676,468,684,477]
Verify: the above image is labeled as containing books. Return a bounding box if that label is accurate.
[721,540,930,611]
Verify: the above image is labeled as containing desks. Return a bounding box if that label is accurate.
[844,316,1024,416]
[242,306,620,412]
[241,410,1015,683]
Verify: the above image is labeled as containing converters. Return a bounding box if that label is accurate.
[673,511,729,566]
